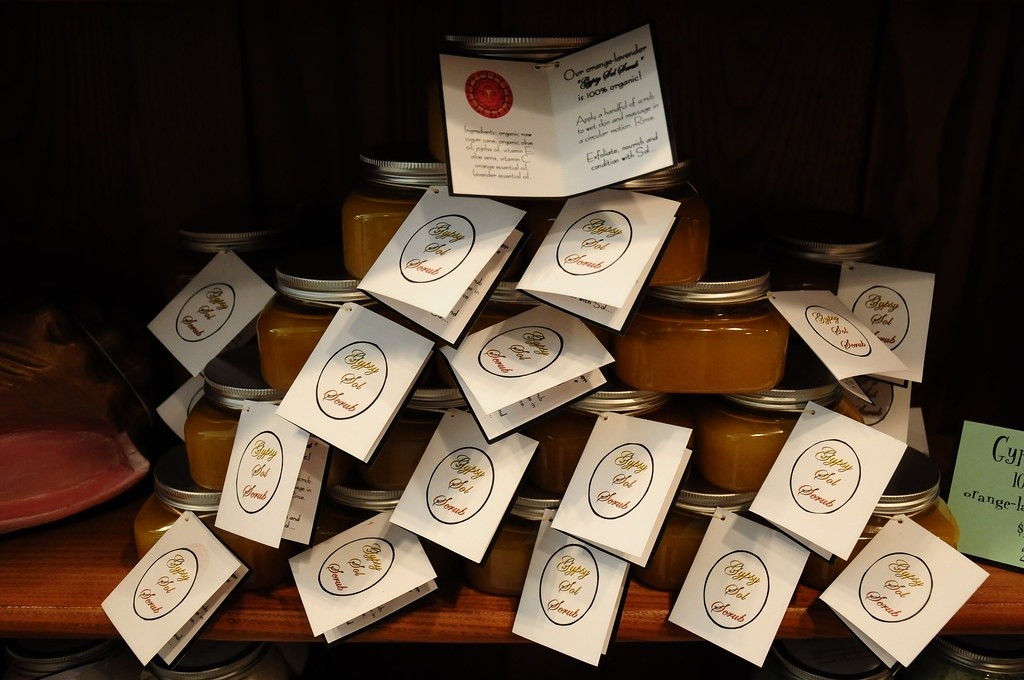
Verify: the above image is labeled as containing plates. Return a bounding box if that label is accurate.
[0,414,149,530]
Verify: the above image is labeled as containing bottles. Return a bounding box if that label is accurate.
[1,30,1024,678]
